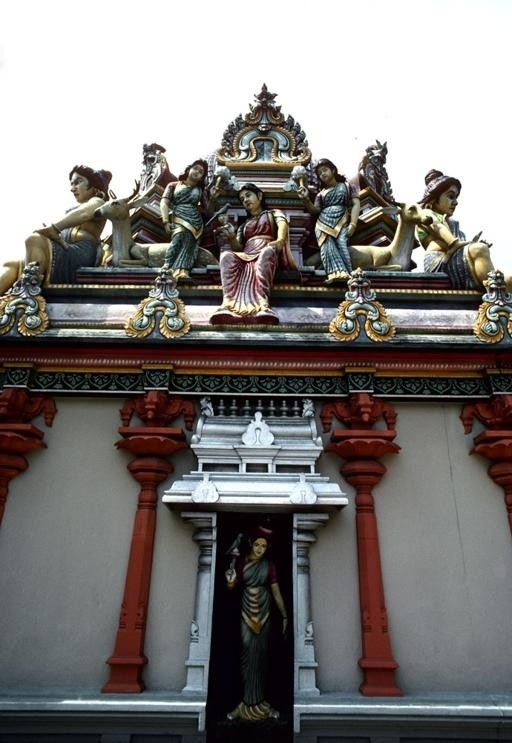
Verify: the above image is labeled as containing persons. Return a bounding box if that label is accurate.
[160,158,222,279]
[223,521,292,721]
[295,156,362,286]
[209,179,290,323]
[414,165,511,303]
[1,163,114,298]
